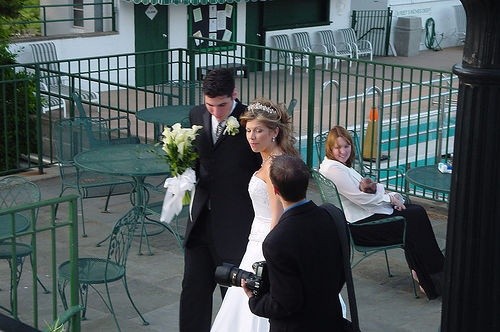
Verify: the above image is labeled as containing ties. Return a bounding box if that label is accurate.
[215,120,227,144]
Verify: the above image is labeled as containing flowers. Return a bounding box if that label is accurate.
[153,120,204,224]
[223,115,241,136]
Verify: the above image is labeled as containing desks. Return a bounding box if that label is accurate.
[406,165,452,193]
[136,105,196,126]
[74,147,184,255]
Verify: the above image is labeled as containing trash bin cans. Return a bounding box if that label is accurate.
[394,15,423,57]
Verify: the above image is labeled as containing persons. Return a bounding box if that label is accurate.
[209,97,304,332]
[241,155,356,332]
[179,69,262,332]
[318,126,445,300]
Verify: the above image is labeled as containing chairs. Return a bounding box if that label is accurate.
[1,42,192,332]
[273,28,373,76]
[311,129,419,300]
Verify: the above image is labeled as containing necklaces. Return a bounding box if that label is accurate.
[260,152,284,167]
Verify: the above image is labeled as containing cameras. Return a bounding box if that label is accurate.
[215,261,268,298]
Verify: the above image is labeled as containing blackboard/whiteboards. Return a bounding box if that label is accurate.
[188,3,238,51]
